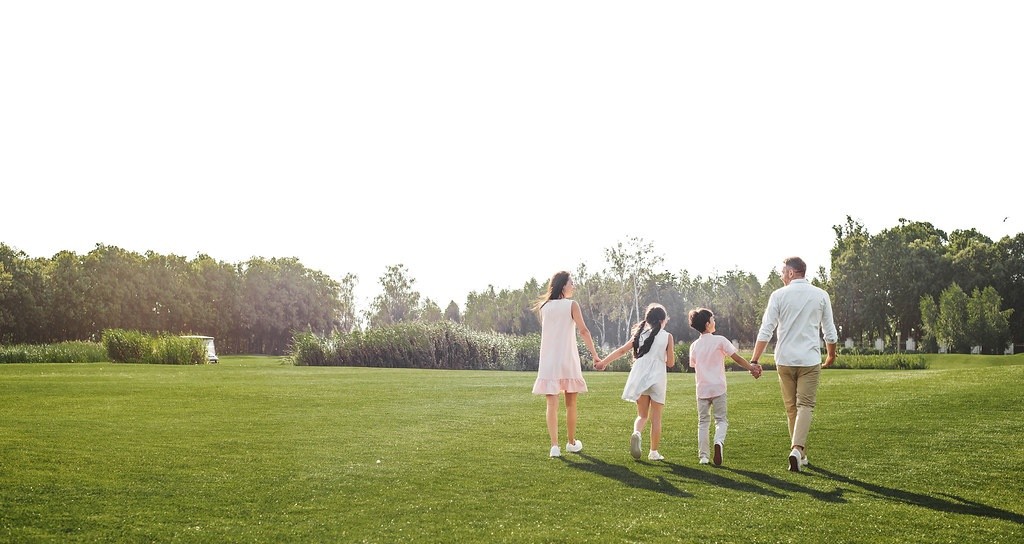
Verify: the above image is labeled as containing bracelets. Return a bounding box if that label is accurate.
[750,361,757,364]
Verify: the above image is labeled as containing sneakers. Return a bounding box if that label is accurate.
[699,456,711,465]
[787,449,803,472]
[630,433,642,459]
[565,438,583,453]
[800,455,808,466]
[713,440,723,465]
[549,445,561,458]
[647,451,666,461]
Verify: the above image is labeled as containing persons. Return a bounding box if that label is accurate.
[747,256,838,471]
[531,270,602,459]
[595,302,677,460]
[686,307,760,466]
